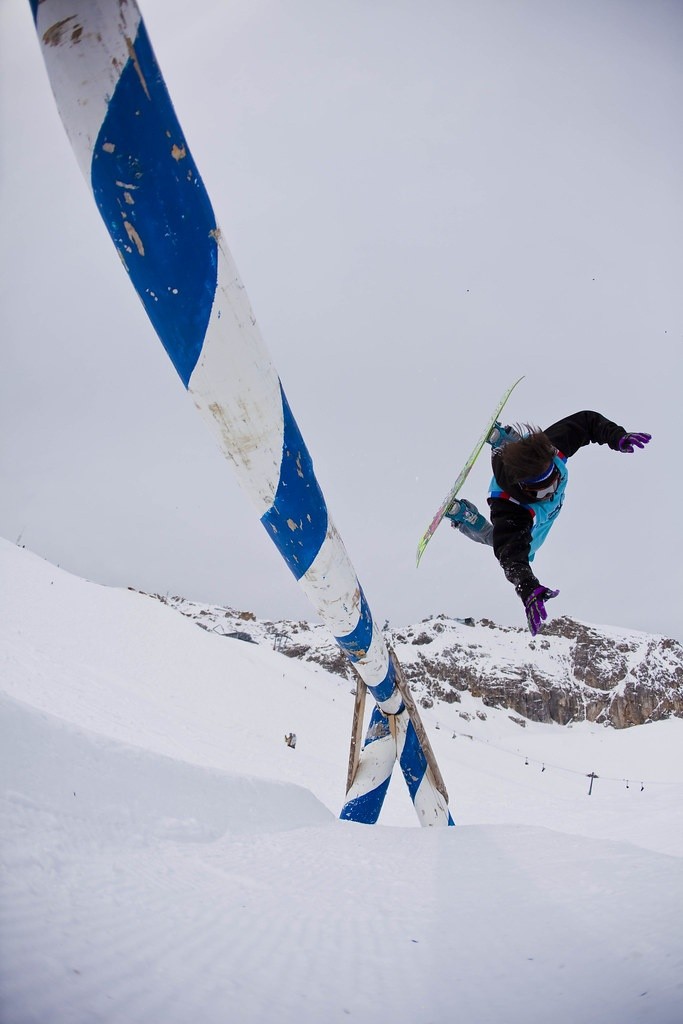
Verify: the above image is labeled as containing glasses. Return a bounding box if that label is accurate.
[522,463,563,500]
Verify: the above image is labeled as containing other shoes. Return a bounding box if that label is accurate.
[489,423,517,447]
[448,500,485,531]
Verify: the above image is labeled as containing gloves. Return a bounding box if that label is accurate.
[516,580,560,636]
[618,432,652,453]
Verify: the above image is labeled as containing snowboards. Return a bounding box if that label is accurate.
[415,373,528,569]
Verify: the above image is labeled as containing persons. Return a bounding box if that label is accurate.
[445,411,652,637]
